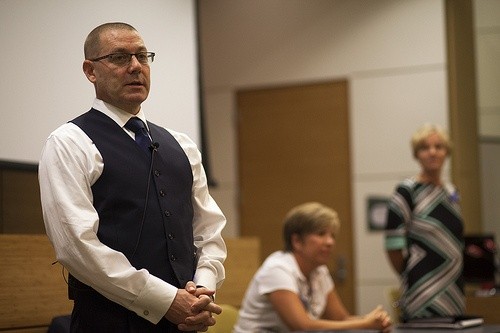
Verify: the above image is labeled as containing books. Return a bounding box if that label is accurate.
[398,314,483,330]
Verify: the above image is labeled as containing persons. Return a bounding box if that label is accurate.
[233,201,393,333]
[38,22,228,333]
[383,126,467,320]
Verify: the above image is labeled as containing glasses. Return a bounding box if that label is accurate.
[89,51,156,65]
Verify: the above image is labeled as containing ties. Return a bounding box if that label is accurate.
[123,117,154,161]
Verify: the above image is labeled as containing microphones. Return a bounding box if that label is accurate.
[154,141,159,148]
